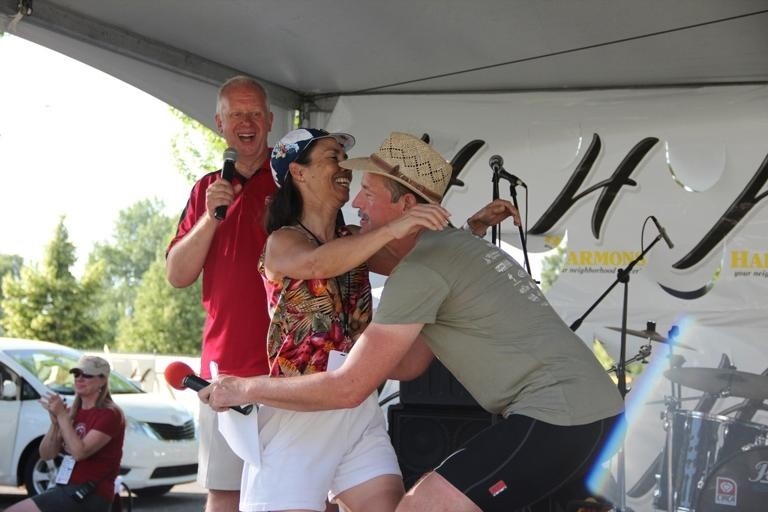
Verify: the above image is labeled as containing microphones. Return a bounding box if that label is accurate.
[215,147,237,219]
[489,152,527,187]
[651,216,675,249]
[164,361,253,416]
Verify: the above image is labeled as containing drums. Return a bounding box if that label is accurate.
[652,408,690,511]
[694,439,768,512]
[678,408,767,512]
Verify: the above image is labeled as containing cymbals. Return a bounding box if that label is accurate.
[604,325,698,352]
[663,366,768,401]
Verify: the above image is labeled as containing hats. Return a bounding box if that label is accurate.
[69,354,110,378]
[270,128,355,188]
[338,132,453,205]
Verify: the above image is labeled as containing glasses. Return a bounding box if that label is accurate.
[74,372,93,378]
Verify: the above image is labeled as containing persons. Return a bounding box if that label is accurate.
[0,355,126,512]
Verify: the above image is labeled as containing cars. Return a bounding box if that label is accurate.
[0,336,202,499]
